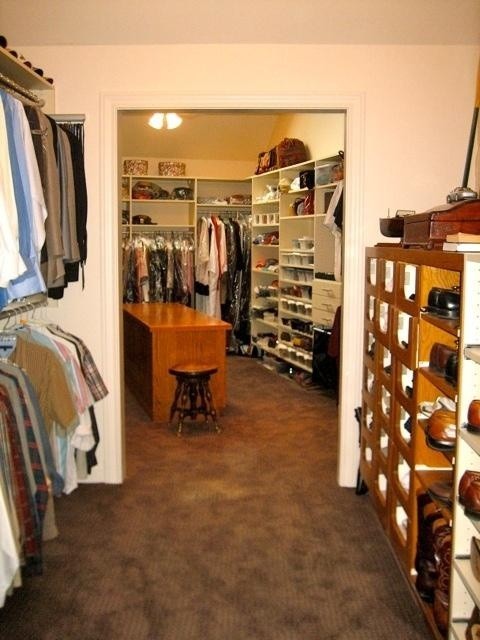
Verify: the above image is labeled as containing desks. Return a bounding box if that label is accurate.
[123,302,233,422]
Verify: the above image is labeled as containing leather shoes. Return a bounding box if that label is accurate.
[429,342,458,388]
[459,470,480,521]
[415,550,449,635]
[428,481,454,513]
[428,287,461,319]
[425,410,456,451]
[467,400,480,433]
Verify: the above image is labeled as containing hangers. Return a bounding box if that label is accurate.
[123,230,195,244]
[1,74,46,109]
[198,210,249,222]
[1,300,52,327]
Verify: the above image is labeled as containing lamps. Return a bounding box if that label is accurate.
[148,112,182,130]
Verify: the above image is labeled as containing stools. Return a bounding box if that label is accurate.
[166,362,224,436]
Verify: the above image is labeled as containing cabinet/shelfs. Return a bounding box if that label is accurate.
[358,246,464,640]
[448,254,479,639]
[251,154,343,375]
[122,175,250,327]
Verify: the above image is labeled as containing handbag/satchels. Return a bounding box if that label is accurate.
[279,170,315,216]
[255,138,306,175]
[331,151,344,181]
[133,181,160,197]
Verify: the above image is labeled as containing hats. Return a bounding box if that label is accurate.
[133,214,157,224]
